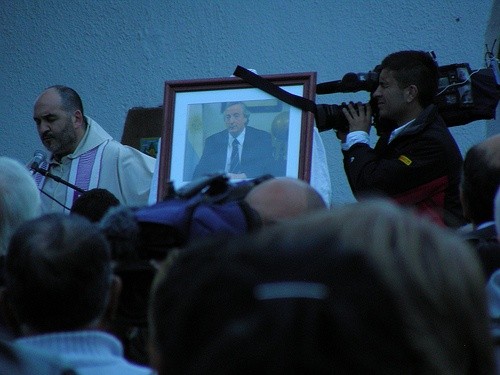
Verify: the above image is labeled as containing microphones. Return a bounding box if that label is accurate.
[28,150,48,176]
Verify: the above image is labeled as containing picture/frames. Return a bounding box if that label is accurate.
[156,71,317,204]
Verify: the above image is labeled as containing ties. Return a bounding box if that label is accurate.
[230,139,242,173]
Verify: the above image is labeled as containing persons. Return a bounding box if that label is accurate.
[24,85,156,223]
[0,134,500,375]
[192,102,272,180]
[341,51,472,230]
